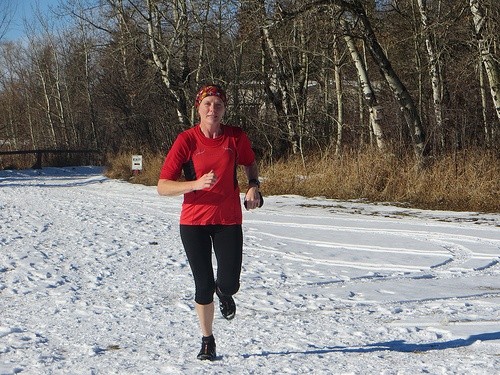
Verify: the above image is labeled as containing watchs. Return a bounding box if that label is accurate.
[248,178,260,188]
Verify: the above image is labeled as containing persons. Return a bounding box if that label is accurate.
[158,84,260,360]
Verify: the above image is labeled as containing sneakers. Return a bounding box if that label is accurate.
[215,284,236,321]
[197,336,218,361]
